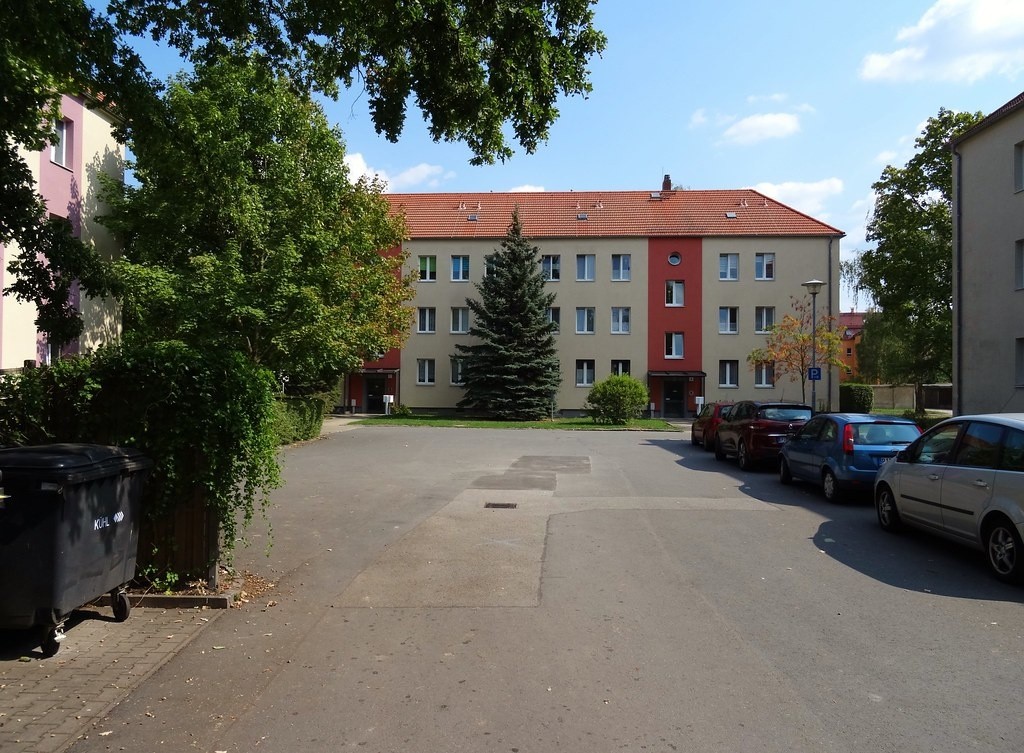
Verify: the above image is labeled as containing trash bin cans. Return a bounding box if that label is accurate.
[0,443,140,656]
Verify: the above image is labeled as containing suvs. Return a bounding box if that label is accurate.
[713,400,814,474]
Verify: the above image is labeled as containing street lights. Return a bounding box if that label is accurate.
[801,277,827,414]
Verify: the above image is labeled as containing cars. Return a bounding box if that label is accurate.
[874,410,1024,588]
[778,412,940,506]
[690,400,735,452]
